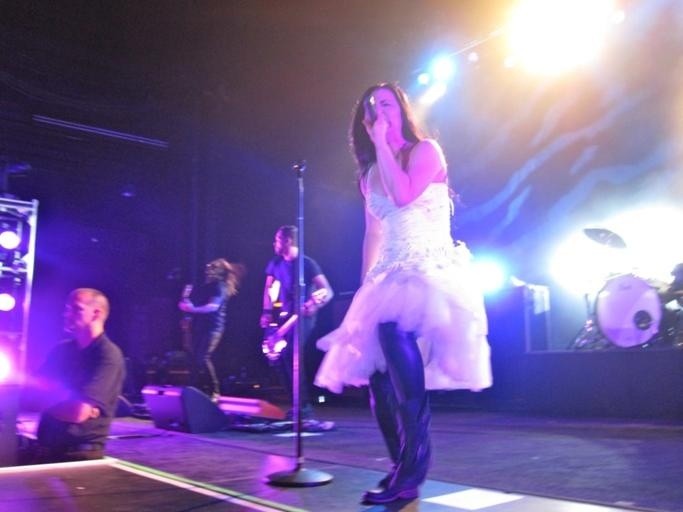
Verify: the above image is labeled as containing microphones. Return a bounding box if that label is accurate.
[364,96,377,124]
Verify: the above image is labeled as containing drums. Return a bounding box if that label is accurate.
[595,274,664,349]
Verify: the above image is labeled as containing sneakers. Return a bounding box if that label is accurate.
[286,402,313,420]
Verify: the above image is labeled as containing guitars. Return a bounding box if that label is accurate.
[178,284,199,354]
[262,288,328,360]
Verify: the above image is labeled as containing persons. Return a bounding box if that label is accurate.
[313,81,492,505]
[260,225,335,420]
[177,256,240,404]
[15,286,126,464]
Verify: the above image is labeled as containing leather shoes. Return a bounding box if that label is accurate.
[362,481,418,502]
[389,451,435,491]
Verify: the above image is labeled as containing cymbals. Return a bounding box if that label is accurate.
[585,229,625,247]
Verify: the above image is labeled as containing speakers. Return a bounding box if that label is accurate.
[484,283,549,352]
[142,385,228,433]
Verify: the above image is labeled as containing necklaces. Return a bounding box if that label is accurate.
[393,141,409,160]
